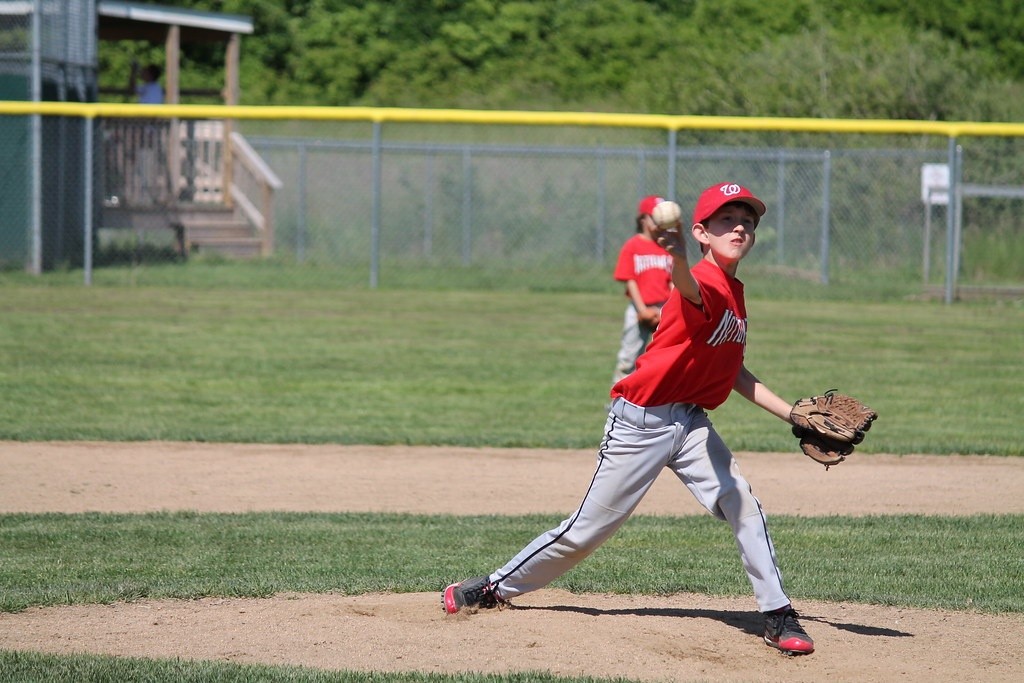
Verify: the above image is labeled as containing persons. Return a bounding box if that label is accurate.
[614,196,674,384]
[137,64,166,164]
[440,182,878,654]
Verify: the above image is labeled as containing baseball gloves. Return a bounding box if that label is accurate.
[789,388,878,471]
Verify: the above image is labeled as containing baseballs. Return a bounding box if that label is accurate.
[651,200,682,229]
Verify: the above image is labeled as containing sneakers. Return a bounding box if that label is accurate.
[762,609,815,653]
[440,576,503,614]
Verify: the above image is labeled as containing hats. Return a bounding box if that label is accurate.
[639,196,665,215]
[694,181,766,224]
